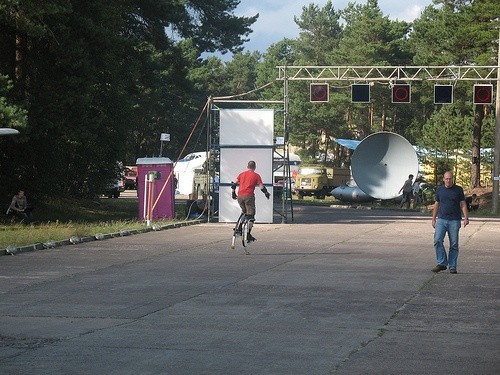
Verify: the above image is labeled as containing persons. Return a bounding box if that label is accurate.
[465,194,479,212]
[6,191,33,225]
[399,174,413,210]
[432,171,469,275]
[231,161,270,243]
[413,178,421,208]
[209,170,219,215]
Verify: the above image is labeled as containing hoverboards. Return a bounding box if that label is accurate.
[232,213,255,249]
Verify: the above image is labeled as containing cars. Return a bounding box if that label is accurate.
[103,173,120,198]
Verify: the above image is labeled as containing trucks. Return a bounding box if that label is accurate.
[292,163,351,200]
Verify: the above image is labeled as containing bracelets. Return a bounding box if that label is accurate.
[465,218,468,220]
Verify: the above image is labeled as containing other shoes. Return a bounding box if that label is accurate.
[432,264,447,272]
[235,228,242,233]
[450,269,456,273]
[247,234,254,240]
